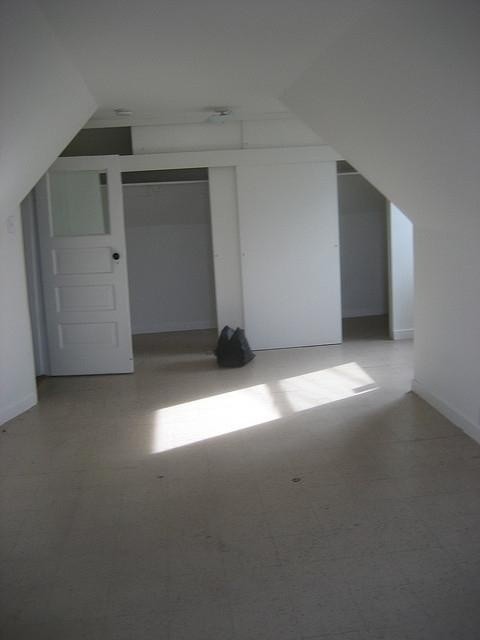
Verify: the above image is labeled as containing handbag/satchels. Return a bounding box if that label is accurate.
[216,325,256,368]
